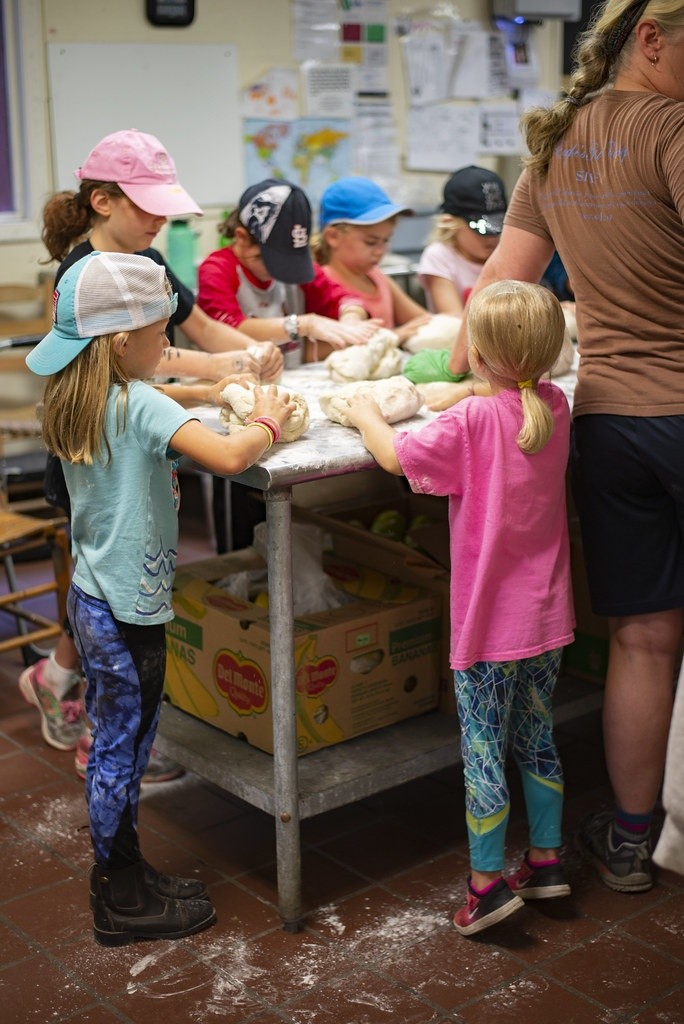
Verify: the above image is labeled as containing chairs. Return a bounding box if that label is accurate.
[0,512,95,729]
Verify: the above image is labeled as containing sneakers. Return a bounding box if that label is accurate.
[76,734,185,784]
[505,855,573,898]
[575,811,653,893]
[20,660,87,752]
[454,867,524,938]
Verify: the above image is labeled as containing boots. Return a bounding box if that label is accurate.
[89,857,217,947]
[88,853,211,918]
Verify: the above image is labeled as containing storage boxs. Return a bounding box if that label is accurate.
[161,474,609,767]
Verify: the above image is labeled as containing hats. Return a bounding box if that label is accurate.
[25,250,180,376]
[241,178,314,283]
[75,130,202,216]
[442,165,508,234]
[319,176,415,232]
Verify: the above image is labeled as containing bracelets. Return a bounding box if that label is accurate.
[341,305,368,320]
[245,414,281,452]
[468,382,476,395]
[287,313,299,341]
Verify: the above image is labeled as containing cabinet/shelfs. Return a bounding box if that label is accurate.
[152,337,606,932]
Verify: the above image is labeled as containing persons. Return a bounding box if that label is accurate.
[20,123,283,784]
[419,164,508,318]
[311,176,437,349]
[197,178,387,558]
[39,252,216,948]
[402,0,684,893]
[331,278,577,935]
